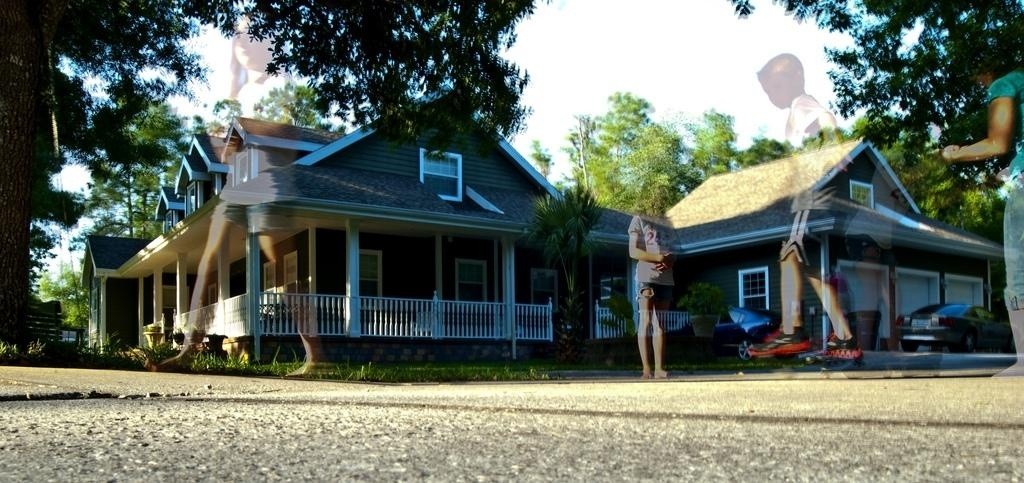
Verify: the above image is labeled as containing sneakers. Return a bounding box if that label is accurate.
[748,324,811,356]
[812,333,862,359]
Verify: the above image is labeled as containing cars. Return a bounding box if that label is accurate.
[894,301,996,350]
[706,306,795,361]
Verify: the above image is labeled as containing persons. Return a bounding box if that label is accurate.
[143,16,330,379]
[744,55,864,365]
[941,55,1024,377]
[629,188,682,378]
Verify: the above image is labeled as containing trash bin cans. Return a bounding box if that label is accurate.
[845,310,882,352]
